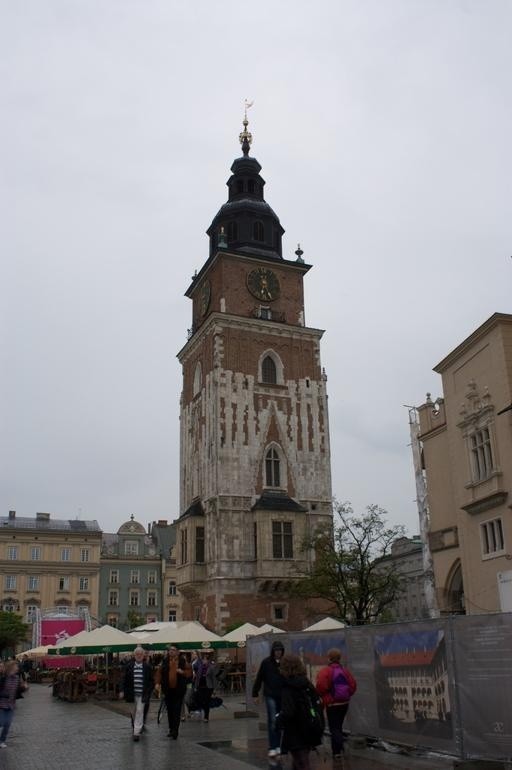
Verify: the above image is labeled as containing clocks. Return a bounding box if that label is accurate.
[247,266,280,302]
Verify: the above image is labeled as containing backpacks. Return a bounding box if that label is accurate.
[321,664,354,702]
[298,684,325,735]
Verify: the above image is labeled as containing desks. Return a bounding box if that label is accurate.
[229,672,246,696]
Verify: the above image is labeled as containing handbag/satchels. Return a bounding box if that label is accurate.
[188,693,200,711]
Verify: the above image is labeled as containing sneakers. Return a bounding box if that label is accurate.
[0,741,8,748]
[267,747,281,756]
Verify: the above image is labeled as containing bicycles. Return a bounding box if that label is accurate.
[158,695,189,723]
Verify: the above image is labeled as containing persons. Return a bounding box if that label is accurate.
[117,646,218,742]
[2,653,31,751]
[251,641,355,770]
[50,666,111,705]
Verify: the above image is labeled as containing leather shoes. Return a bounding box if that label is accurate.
[133,736,139,742]
[167,731,178,739]
